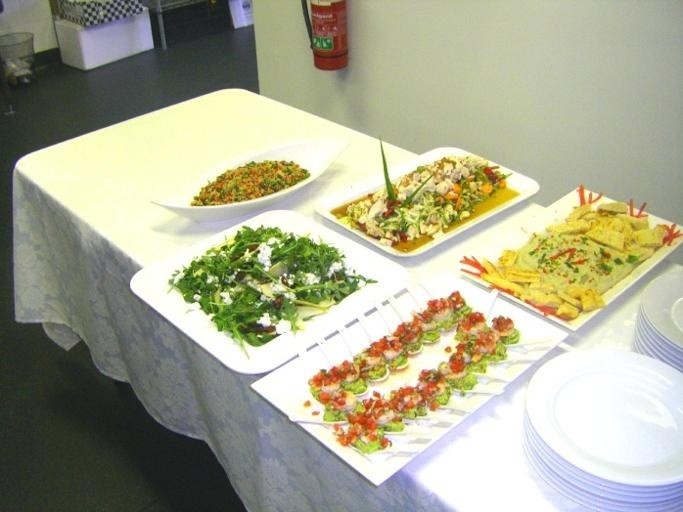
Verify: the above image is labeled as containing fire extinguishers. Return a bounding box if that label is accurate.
[301,0,348,70]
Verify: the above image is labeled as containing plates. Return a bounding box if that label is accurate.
[521,345,682,511]
[127,208,408,377]
[150,141,338,222]
[248,268,568,489]
[628,265,683,372]
[456,185,682,332]
[311,144,540,260]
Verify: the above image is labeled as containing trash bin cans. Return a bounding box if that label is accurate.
[0,32,33,90]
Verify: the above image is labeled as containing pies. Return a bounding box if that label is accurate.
[477,197,669,318]
